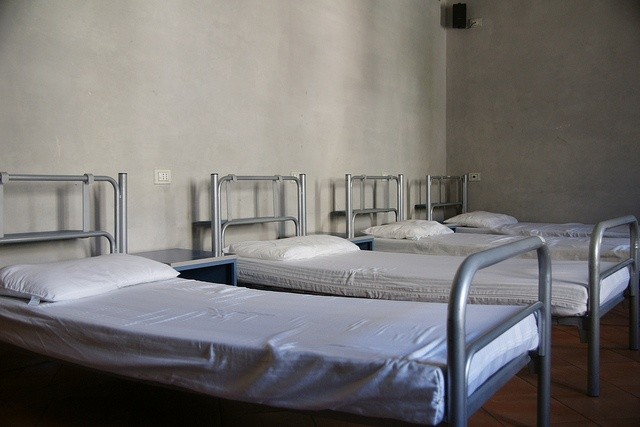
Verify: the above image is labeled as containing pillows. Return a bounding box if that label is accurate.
[221,235,361,260]
[0,253,182,303]
[442,210,518,229]
[360,219,454,241]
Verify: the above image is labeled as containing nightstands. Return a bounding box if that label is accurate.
[278,230,376,252]
[128,249,238,287]
[437,221,457,233]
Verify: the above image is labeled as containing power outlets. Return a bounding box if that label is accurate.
[154,168,172,185]
[468,172,481,182]
[469,17,483,28]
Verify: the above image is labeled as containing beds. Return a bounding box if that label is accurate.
[343,171,640,272]
[415,173,640,237]
[209,171,640,399]
[0,172,554,427]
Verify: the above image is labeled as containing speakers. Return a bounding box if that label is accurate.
[452,3,467,30]
